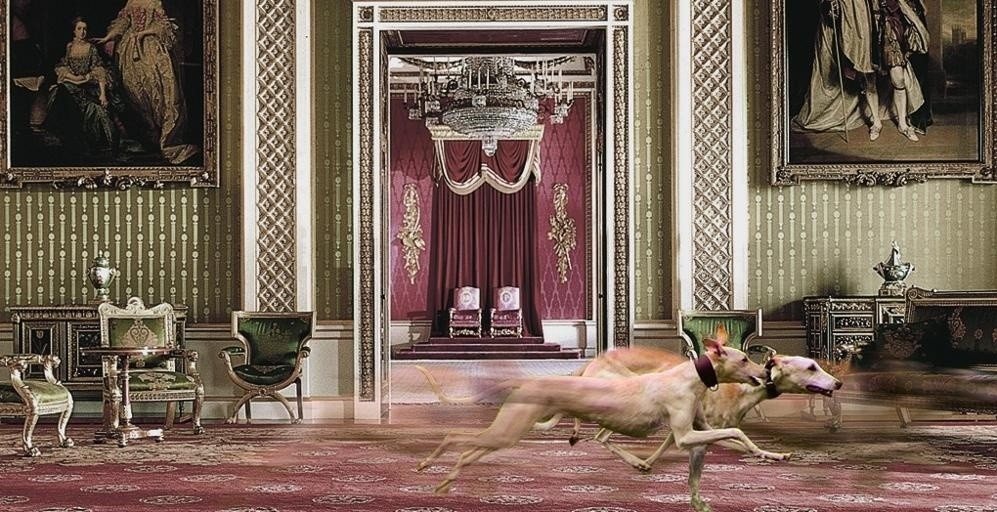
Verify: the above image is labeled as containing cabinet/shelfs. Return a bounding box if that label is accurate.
[3,304,191,424]
[801,292,909,421]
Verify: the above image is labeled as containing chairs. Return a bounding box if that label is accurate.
[676,309,776,422]
[97,297,318,437]
[447,285,524,339]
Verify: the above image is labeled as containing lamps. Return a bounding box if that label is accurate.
[402,52,575,158]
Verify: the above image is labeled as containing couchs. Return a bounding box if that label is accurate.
[819,286,997,429]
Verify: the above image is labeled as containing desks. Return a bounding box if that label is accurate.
[79,345,174,447]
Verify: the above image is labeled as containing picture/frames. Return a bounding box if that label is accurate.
[766,0,997,187]
[1,0,222,192]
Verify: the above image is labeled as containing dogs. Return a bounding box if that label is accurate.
[415,337,792,492]
[530,345,842,512]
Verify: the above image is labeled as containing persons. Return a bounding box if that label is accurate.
[86,0,207,165]
[789,0,945,144]
[52,17,144,168]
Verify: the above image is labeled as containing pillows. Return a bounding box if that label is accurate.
[864,316,940,373]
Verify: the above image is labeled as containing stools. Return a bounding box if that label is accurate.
[0,352,75,458]
